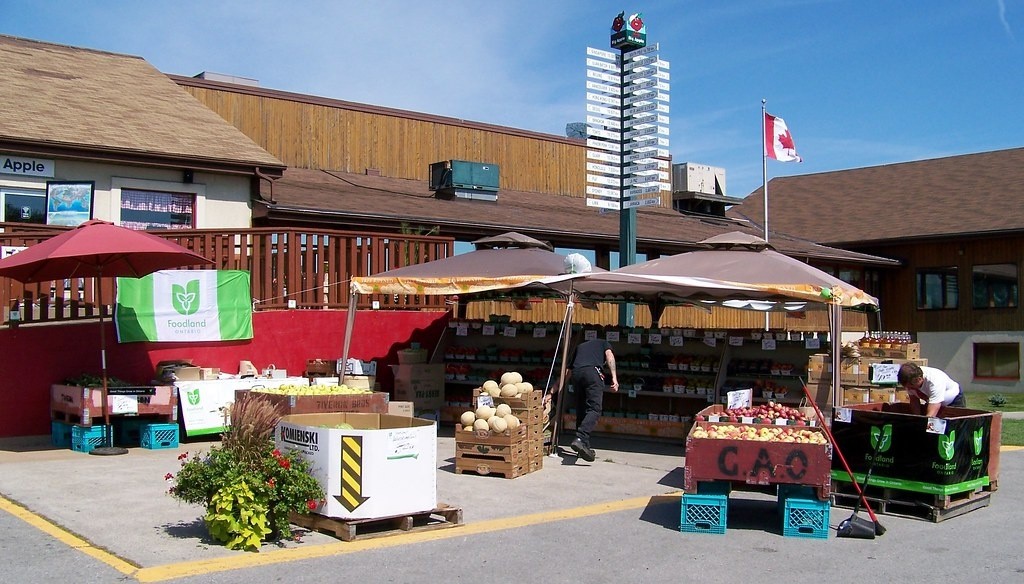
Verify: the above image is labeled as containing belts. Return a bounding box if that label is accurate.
[582,365,600,368]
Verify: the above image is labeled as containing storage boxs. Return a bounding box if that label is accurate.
[777,486,830,538]
[680,482,732,533]
[616,355,719,372]
[139,423,179,449]
[262,369,287,378]
[751,329,829,343]
[72,424,113,452]
[155,359,222,381]
[726,362,792,375]
[830,402,1001,495]
[275,413,438,521]
[120,419,157,445]
[804,355,832,430]
[234,389,390,418]
[307,360,337,378]
[394,364,446,415]
[445,348,563,364]
[389,402,414,418]
[719,384,789,398]
[542,397,557,433]
[455,387,544,479]
[604,374,715,394]
[51,420,74,450]
[449,313,728,343]
[336,358,376,377]
[840,343,927,405]
[602,408,691,422]
[569,408,576,413]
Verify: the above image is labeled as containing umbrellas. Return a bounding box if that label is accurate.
[0,219,217,450]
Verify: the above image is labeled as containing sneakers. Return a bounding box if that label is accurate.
[571,437,596,462]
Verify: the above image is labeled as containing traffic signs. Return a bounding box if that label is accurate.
[586,41,673,215]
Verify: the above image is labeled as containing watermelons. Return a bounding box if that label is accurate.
[318,422,354,429]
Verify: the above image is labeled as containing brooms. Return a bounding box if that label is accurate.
[797,374,886,536]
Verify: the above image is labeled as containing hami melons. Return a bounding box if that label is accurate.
[483,371,533,398]
[461,402,520,434]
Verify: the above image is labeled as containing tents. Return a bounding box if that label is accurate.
[548,230,884,456]
[339,233,612,385]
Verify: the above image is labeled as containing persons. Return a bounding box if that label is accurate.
[545,339,619,462]
[897,362,966,418]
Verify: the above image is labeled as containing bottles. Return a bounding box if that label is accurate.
[861,331,912,348]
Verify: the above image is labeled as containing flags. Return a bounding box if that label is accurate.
[764,112,803,163]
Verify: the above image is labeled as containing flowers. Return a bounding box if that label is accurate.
[163,386,327,548]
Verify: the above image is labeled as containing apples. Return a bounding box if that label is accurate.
[708,401,807,421]
[249,384,372,395]
[692,425,828,444]
